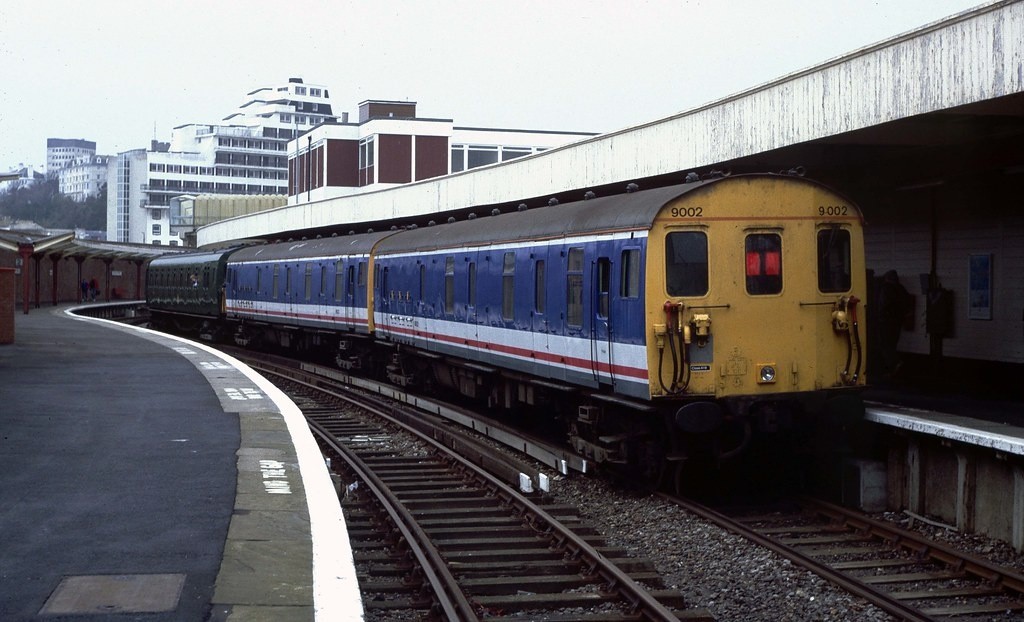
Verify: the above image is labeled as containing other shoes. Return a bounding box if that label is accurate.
[93,298,96,302]
[82,301,86,304]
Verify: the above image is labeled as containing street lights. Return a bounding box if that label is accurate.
[308,135,312,201]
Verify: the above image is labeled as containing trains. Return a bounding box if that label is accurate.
[145,172,869,501]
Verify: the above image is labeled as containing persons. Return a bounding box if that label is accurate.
[867,269,910,373]
[88,276,99,302]
[190,275,197,285]
[80,279,89,302]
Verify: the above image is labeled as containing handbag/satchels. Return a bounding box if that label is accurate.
[96,289,100,295]
